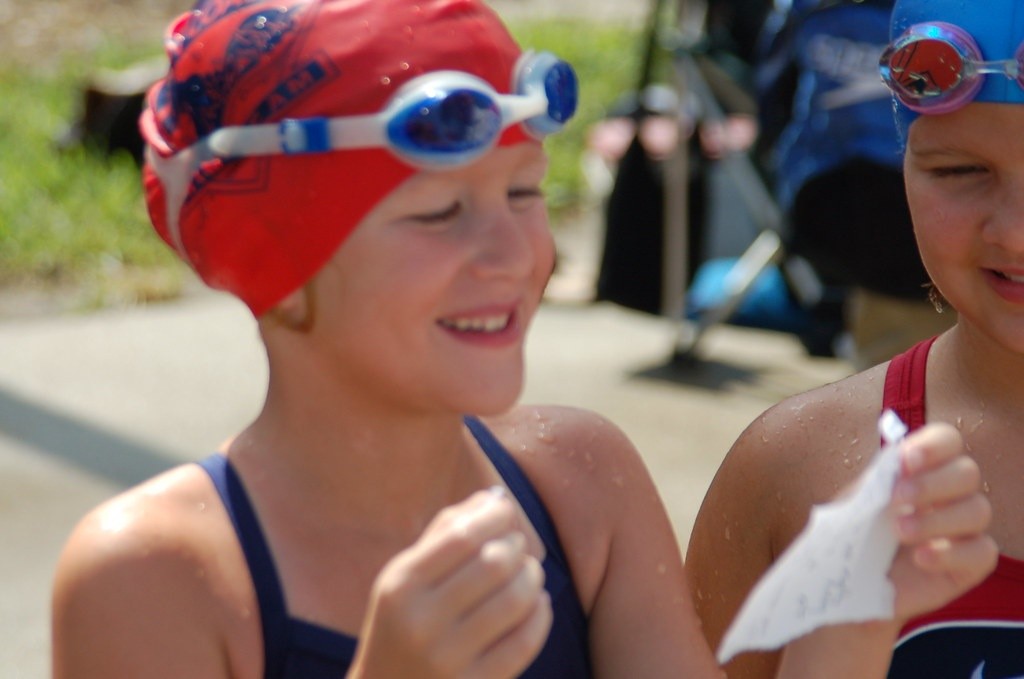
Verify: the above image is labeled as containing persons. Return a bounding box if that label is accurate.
[686,0,1024,679]
[48,0,997,679]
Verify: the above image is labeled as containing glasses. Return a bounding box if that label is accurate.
[878,21,1024,115]
[144,49,579,269]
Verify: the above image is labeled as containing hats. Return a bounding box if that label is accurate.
[889,0,1024,154]
[139,0,541,319]
